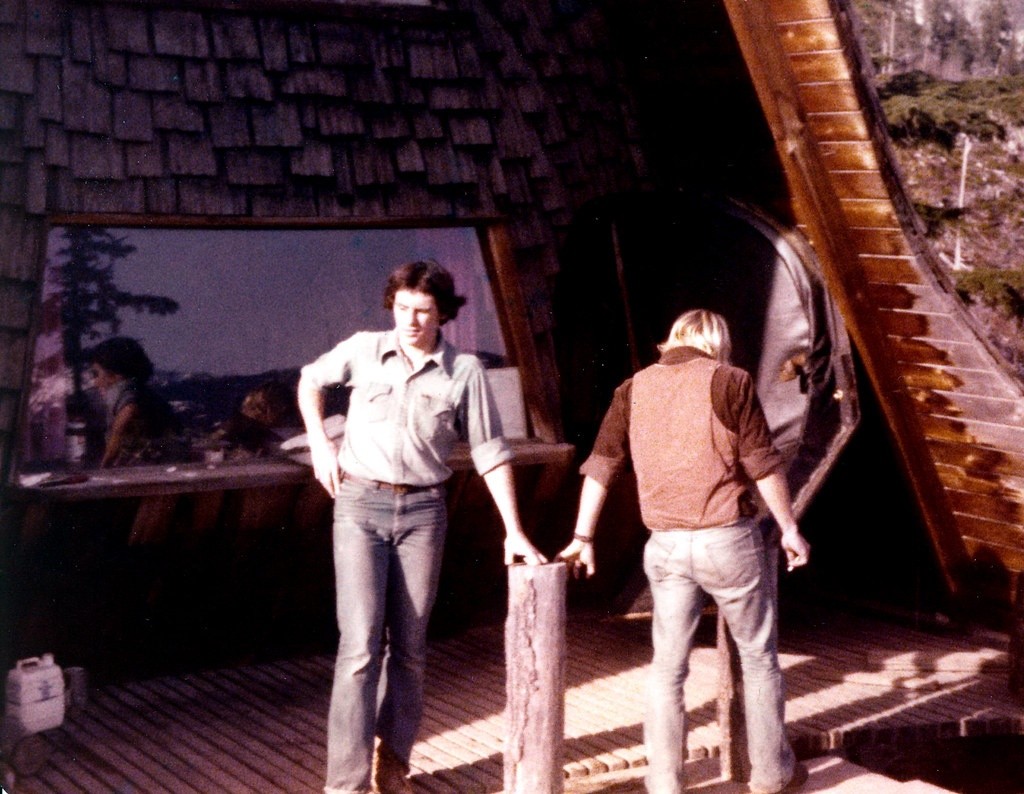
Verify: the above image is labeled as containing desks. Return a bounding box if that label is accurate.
[16,439,575,547]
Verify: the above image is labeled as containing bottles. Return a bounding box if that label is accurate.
[62,666,93,709]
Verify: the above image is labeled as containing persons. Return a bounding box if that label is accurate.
[551,311,813,794]
[295,259,551,794]
[89,336,191,468]
[209,388,284,456]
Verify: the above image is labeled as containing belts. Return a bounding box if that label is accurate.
[338,469,426,494]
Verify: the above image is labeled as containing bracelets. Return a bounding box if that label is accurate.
[573,532,592,542]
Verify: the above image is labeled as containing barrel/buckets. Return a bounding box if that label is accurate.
[4,653,65,734]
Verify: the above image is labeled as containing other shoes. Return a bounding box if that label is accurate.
[786,764,808,786]
